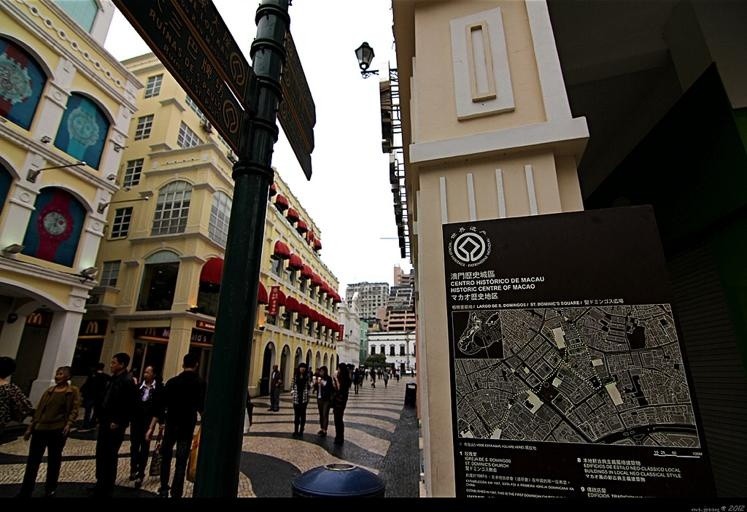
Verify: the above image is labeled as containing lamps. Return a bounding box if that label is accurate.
[26,162,87,183]
[355,42,397,82]
[80,267,99,284]
[4,244,25,254]
[97,196,149,214]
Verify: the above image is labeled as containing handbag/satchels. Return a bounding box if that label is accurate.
[149,449,163,476]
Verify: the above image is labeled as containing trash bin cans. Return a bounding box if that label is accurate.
[260,377,269,396]
[290,463,386,498]
[404,383,417,409]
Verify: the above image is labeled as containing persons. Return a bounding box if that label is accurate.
[0,347,254,502]
[268,359,415,437]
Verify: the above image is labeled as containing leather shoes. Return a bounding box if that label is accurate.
[159,489,169,498]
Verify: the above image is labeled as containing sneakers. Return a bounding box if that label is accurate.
[318,430,327,437]
[298,433,303,440]
[292,432,296,437]
[267,409,278,411]
[129,470,145,481]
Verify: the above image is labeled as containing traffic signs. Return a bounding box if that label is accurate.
[277,30,316,181]
[116,0,251,150]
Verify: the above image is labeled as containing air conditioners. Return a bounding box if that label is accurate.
[203,119,213,131]
